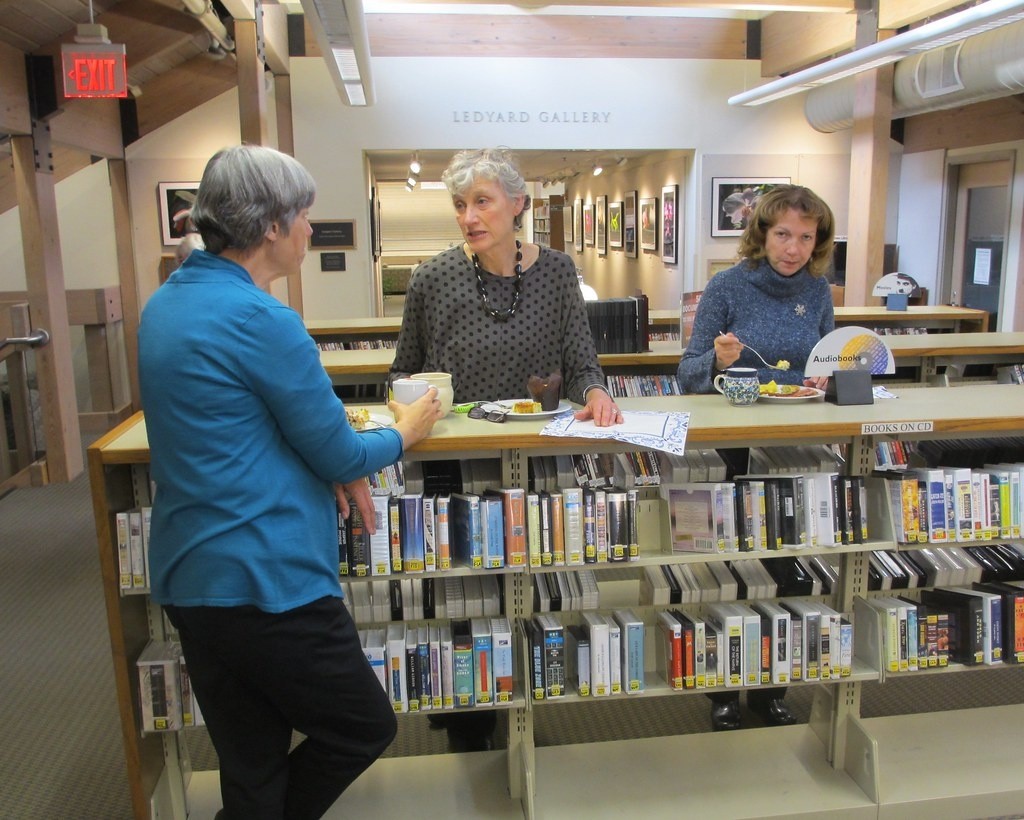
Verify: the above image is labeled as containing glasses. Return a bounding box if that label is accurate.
[468,407,508,423]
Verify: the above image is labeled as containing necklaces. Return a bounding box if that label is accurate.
[472,240,523,320]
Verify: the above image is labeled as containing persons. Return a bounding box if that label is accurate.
[676,184,836,731]
[389,147,624,752]
[137,145,443,820]
[897,273,916,297]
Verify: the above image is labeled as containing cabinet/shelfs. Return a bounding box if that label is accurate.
[88,306,1023,819]
[533,194,565,253]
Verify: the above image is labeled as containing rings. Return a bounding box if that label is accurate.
[611,407,617,413]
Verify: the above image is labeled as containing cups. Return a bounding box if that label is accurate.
[393,380,440,423]
[410,372,453,418]
[713,367,759,407]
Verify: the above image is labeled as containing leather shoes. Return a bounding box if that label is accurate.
[710,695,740,731]
[750,697,797,727]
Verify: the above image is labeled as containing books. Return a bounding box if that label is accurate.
[137,639,205,731]
[116,505,152,588]
[317,340,398,351]
[874,328,927,335]
[336,440,1024,713]
[607,375,683,397]
[649,333,679,341]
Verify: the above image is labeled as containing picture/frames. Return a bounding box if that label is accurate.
[574,199,583,252]
[624,190,637,258]
[584,204,595,244]
[661,185,679,263]
[158,180,201,244]
[641,197,658,249]
[711,175,792,236]
[563,206,573,242]
[608,201,623,247]
[595,195,607,255]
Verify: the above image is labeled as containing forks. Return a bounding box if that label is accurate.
[719,330,789,370]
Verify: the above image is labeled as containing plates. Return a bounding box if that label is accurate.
[354,413,396,431]
[480,399,571,419]
[758,384,826,403]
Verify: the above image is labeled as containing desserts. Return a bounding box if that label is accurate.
[514,401,543,413]
[781,384,799,393]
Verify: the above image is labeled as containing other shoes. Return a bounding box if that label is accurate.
[474,737,492,751]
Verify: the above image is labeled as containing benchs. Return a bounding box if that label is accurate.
[382,262,419,294]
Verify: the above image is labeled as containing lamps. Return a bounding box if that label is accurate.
[539,167,581,188]
[405,150,425,192]
[729,1,1024,108]
[589,158,603,175]
[612,153,628,167]
[301,0,376,107]
